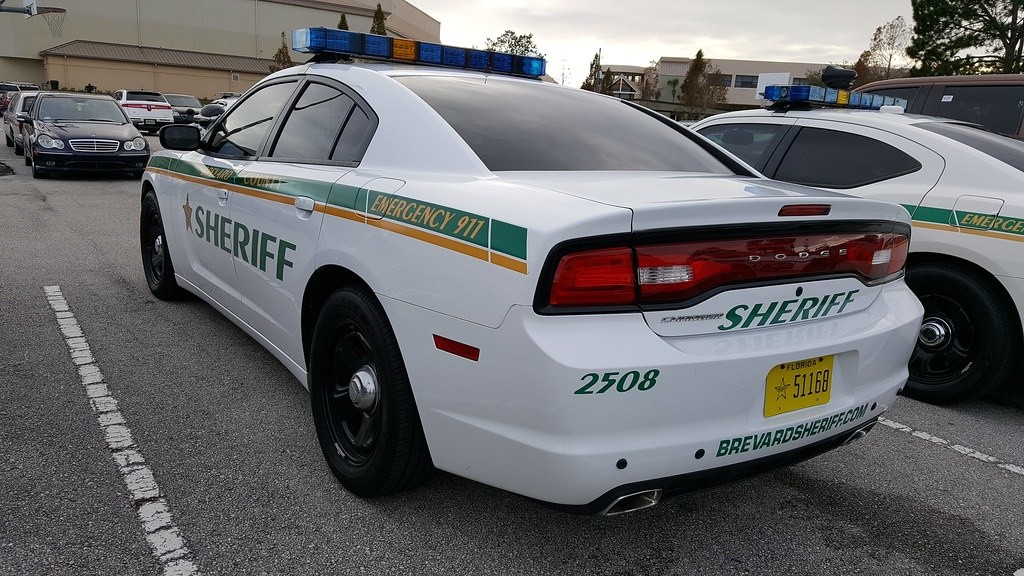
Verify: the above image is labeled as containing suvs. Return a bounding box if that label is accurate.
[0,81,40,99]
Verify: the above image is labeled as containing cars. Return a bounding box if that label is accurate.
[208,92,243,114]
[0,91,17,117]
[164,93,211,130]
[689,84,1023,405]
[139,27,925,517]
[17,94,151,180]
[113,90,174,134]
[3,91,48,156]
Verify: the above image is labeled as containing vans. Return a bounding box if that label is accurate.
[852,74,1024,140]
